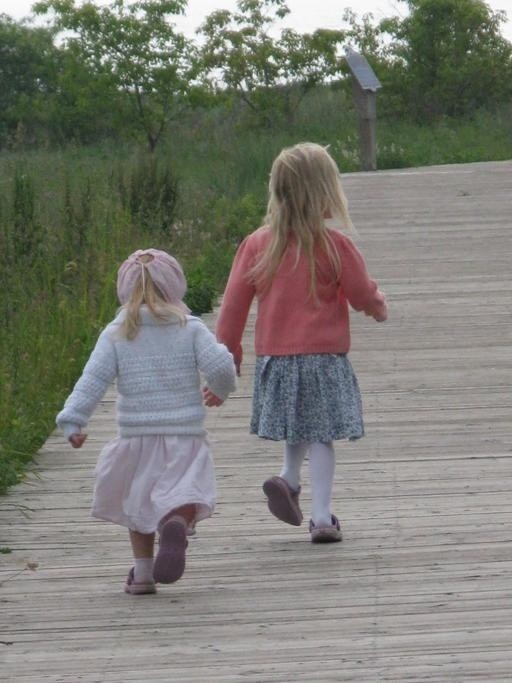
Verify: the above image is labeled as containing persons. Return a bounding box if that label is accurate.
[56,248,238,595]
[216,143,389,541]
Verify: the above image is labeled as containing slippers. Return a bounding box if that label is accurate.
[124,566,156,594]
[309,513,342,542]
[263,475,304,525]
[152,515,188,584]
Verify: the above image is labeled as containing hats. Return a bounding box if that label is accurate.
[115,248,189,308]
